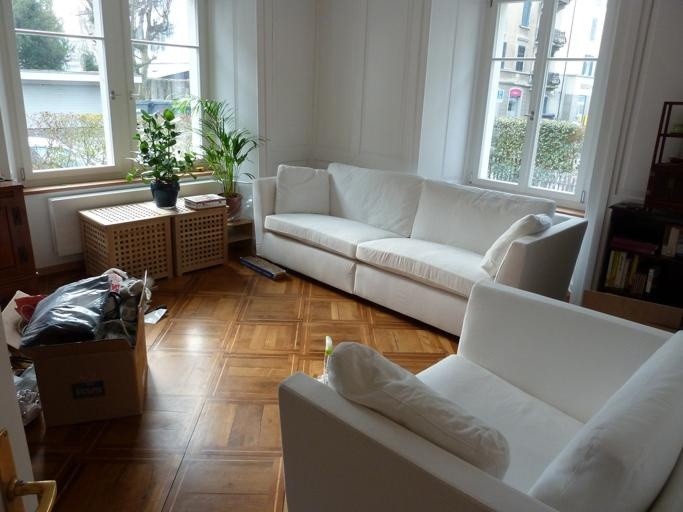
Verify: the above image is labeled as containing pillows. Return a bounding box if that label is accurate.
[323,341,508,478]
[272,163,333,216]
[481,210,551,276]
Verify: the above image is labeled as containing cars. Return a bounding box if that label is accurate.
[136,99,184,131]
[27,134,106,170]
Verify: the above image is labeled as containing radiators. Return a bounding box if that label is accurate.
[47,177,221,258]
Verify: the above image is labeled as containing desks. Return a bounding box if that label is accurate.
[75,194,230,282]
[226,216,253,260]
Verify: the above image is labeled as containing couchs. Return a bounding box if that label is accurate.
[244,156,588,337]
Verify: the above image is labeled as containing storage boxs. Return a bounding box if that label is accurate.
[1,269,157,428]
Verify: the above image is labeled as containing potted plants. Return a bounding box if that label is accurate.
[128,109,188,210]
[195,95,260,220]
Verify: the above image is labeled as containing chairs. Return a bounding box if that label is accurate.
[274,277,682,510]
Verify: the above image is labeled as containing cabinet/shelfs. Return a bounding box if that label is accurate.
[0,178,41,302]
[641,102,682,207]
[596,199,682,309]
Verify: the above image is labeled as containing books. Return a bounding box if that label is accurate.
[601,250,660,298]
[610,220,683,260]
